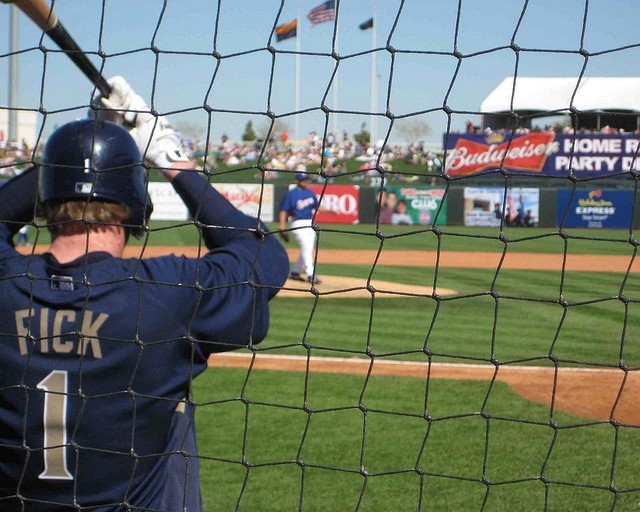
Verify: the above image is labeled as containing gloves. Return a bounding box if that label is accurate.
[90,78,148,122]
[125,109,190,171]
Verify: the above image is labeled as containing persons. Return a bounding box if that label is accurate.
[374,189,388,224]
[378,192,398,224]
[1,136,43,177]
[324,131,445,180]
[278,129,322,172]
[279,173,322,284]
[16,225,31,248]
[525,210,535,227]
[504,207,514,226]
[494,202,502,218]
[0,75,289,511]
[181,126,277,180]
[450,118,640,136]
[513,208,525,226]
[390,200,414,225]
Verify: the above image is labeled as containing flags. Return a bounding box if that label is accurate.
[274,18,297,43]
[359,17,373,31]
[307,0,335,28]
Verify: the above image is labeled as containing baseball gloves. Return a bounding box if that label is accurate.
[278,230,290,242]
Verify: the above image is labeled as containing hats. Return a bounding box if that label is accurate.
[294,172,310,181]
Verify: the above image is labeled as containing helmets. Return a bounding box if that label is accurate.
[35,120,148,225]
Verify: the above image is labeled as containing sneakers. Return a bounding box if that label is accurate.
[307,276,322,283]
[289,273,304,281]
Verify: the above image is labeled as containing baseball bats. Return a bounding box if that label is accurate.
[1,0,136,128]
